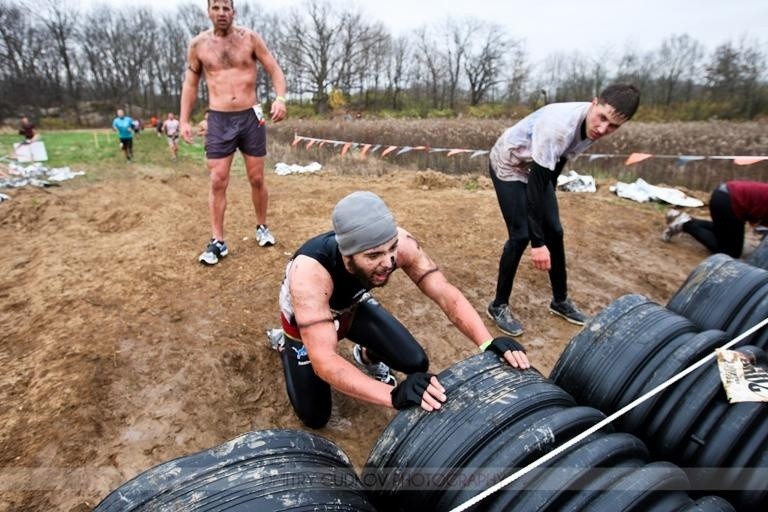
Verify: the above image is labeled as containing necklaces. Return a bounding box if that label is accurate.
[178,0,289,266]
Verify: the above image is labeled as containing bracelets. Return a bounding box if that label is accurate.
[478,336,497,353]
[275,95,286,103]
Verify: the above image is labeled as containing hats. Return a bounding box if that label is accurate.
[332,190,399,257]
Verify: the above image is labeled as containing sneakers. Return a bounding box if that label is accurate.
[662,208,692,243]
[197,238,228,266]
[265,329,285,350]
[351,343,398,387]
[486,299,525,337]
[254,225,276,247]
[547,298,589,326]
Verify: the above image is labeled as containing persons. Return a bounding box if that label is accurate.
[112,108,209,165]
[661,179,768,258]
[485,82,642,336]
[19,116,39,144]
[265,189,530,429]
